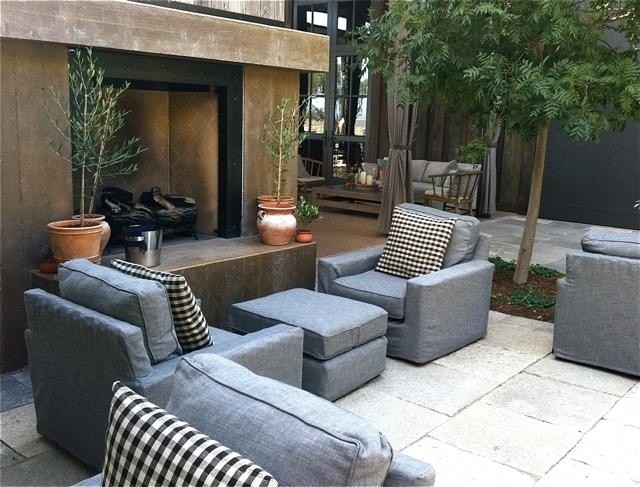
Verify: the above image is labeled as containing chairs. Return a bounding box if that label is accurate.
[318,203,495,365]
[424,171,483,216]
[296,158,325,200]
[550,251,640,380]
[73,424,436,487]
[25,288,305,474]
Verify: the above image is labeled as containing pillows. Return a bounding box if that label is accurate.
[374,206,455,278]
[581,229,640,259]
[436,160,482,198]
[102,378,282,487]
[297,154,311,179]
[106,258,213,353]
[376,158,389,180]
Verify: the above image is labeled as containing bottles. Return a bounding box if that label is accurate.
[353,163,374,185]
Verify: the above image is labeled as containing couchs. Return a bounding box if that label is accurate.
[356,158,482,214]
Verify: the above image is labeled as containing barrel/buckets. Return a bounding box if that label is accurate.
[121,223,164,268]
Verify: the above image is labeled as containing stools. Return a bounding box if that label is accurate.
[228,285,388,402]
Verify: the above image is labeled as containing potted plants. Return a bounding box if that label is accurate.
[344,173,356,191]
[38,45,150,272]
[256,92,320,247]
